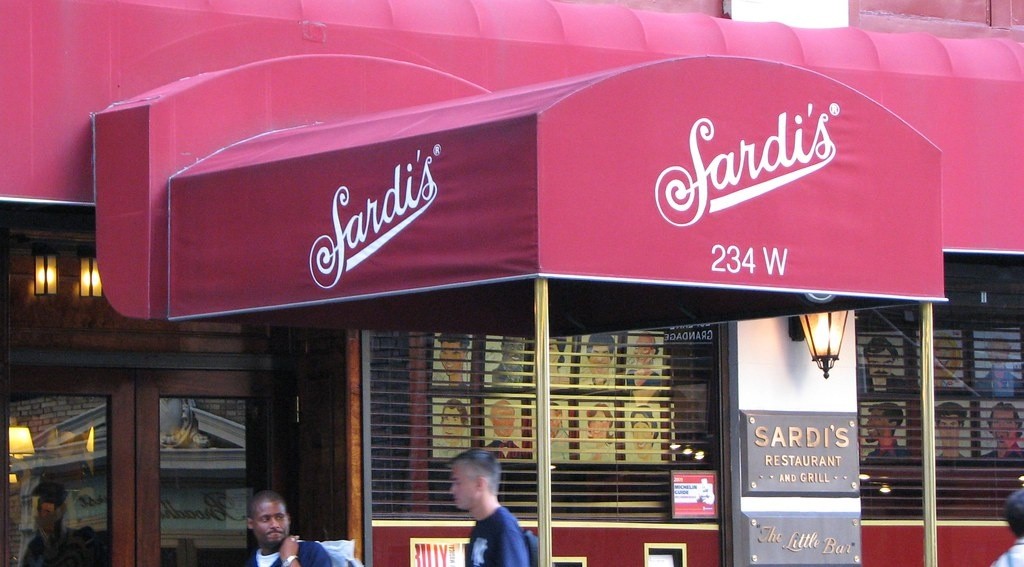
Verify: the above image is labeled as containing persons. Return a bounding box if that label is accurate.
[449,449,531,567]
[242,491,332,567]
[989,488,1024,567]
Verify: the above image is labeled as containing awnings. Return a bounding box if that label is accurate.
[167,53,949,567]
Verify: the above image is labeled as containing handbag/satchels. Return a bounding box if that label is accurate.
[296,539,365,567]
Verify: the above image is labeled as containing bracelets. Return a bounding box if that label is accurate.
[281,555,299,567]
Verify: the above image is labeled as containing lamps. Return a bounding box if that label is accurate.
[76,245,103,297]
[788,311,848,380]
[31,241,61,297]
[10,426,35,459]
